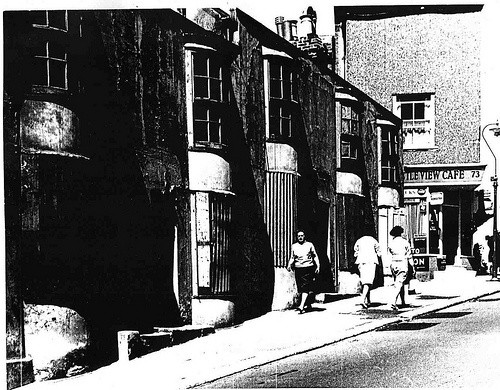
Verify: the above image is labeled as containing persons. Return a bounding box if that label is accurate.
[286,231,321,314]
[353,224,382,307]
[387,226,416,311]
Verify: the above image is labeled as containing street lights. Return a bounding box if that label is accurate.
[482,119,500,282]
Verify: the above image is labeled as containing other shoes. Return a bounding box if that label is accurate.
[360,300,371,308]
[296,305,312,314]
[390,302,412,314]
